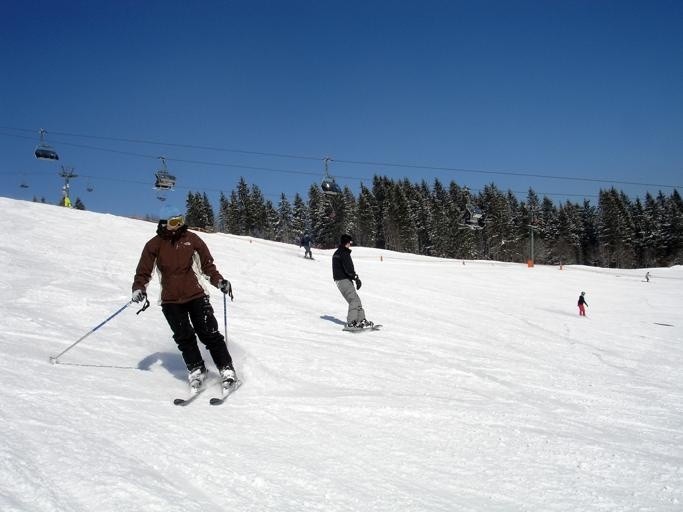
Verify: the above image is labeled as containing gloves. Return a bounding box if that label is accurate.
[220,279,230,294]
[355,278,361,290]
[132,290,145,302]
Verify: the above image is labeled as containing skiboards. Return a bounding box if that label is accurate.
[343,324,382,331]
[173,376,242,406]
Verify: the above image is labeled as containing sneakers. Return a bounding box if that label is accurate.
[347,319,373,328]
[188,366,209,388]
[220,366,238,388]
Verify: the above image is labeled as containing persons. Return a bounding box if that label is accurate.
[331,233,374,328]
[645,272,651,282]
[299,231,315,259]
[130,204,238,389]
[577,291,588,316]
[63,192,72,208]
[321,178,342,201]
[74,196,84,210]
[460,203,486,228]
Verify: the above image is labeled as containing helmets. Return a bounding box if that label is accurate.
[159,204,183,220]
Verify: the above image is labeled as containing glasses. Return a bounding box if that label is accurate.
[168,216,184,227]
